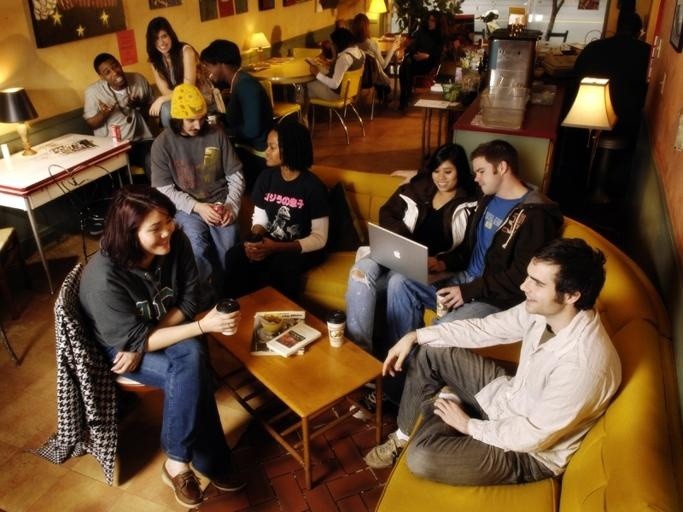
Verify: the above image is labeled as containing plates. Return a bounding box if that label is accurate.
[432,74,455,85]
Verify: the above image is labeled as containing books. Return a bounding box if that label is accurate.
[266,322,322,358]
[248,311,306,356]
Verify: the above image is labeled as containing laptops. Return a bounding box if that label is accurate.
[367,221,457,288]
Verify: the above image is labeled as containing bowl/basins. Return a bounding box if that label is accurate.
[261,314,282,331]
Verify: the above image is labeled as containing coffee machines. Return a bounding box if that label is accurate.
[481,28,542,106]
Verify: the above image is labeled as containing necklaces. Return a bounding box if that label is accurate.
[107,85,134,126]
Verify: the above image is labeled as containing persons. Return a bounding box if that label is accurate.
[568,12,652,166]
[79,184,246,508]
[83,52,152,174]
[150,85,247,299]
[307,8,445,114]
[365,239,622,485]
[345,142,480,350]
[225,123,331,297]
[200,40,274,193]
[350,140,563,420]
[146,18,213,129]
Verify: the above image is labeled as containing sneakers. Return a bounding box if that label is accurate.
[161,461,204,508]
[190,458,247,492]
[365,433,409,468]
[349,391,392,421]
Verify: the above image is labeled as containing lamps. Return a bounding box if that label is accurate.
[561,76,618,146]
[253,32,273,66]
[0,87,40,155]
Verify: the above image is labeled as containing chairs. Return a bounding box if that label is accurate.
[254,48,378,145]
[35,263,165,490]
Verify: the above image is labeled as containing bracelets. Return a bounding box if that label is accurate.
[196,320,208,339]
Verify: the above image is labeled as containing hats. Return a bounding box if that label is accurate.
[171,84,207,120]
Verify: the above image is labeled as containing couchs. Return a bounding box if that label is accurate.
[235,167,682,512]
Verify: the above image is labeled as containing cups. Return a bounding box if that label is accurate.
[215,298,239,337]
[1,144,10,161]
[326,310,346,347]
[246,234,265,262]
[394,35,401,40]
[109,124,120,143]
[454,67,462,82]
[435,293,449,320]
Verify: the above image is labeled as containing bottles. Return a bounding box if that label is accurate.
[393,41,403,63]
[532,53,546,91]
[477,39,482,51]
[483,53,488,72]
[478,57,482,72]
